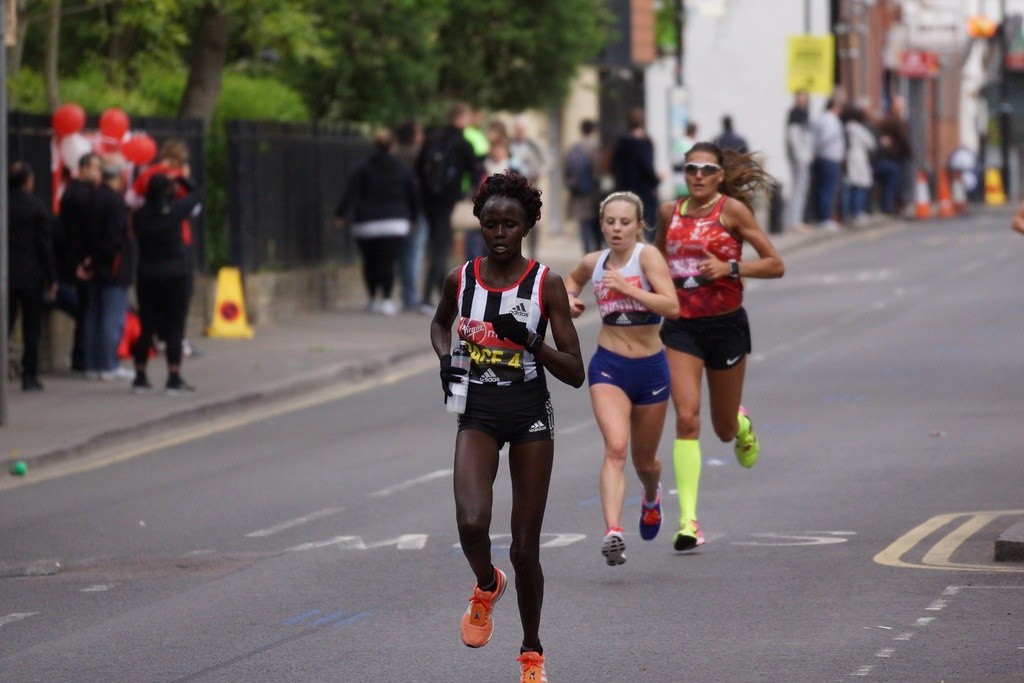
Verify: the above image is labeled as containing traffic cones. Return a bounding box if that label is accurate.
[205,267,253,340]
[952,173,968,216]
[915,172,932,220]
[982,168,1004,205]
[937,169,954,217]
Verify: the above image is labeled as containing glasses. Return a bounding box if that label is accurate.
[683,163,722,177]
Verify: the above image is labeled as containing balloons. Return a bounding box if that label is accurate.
[51,104,158,183]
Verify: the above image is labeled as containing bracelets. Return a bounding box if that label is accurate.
[728,259,738,279]
[567,291,577,297]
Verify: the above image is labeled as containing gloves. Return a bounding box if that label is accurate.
[492,315,529,345]
[440,355,467,404]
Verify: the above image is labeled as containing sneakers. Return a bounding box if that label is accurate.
[673,524,706,551]
[601,530,627,568]
[735,405,758,468]
[517,644,547,683]
[461,566,508,649]
[638,484,661,541]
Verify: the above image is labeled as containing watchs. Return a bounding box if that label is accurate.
[525,333,543,353]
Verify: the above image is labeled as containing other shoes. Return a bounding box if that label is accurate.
[101,366,135,381]
[132,377,154,392]
[382,301,397,316]
[166,379,196,397]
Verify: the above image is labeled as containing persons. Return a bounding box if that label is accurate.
[330,85,1024,319]
[4,138,206,396]
[428,171,584,683]
[653,142,786,549]
[555,191,682,567]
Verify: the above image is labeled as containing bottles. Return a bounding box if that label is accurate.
[445,340,471,414]
